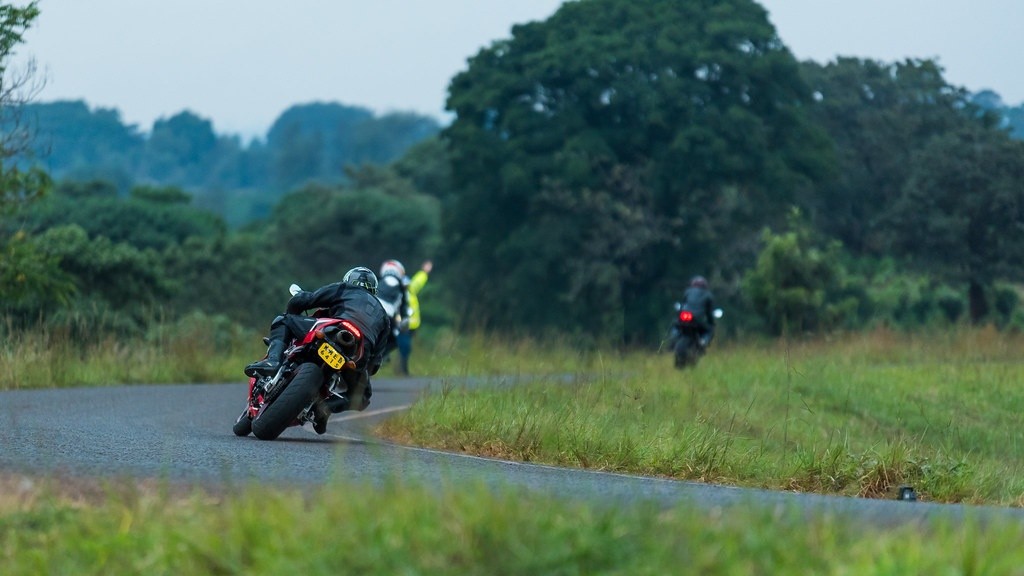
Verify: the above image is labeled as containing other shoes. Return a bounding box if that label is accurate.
[668,335,676,350]
[699,339,706,355]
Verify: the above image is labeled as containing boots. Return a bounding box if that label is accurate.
[244,339,286,377]
[313,398,345,434]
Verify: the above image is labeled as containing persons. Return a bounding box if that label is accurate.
[373,259,434,375]
[663,277,718,356]
[243,266,391,435]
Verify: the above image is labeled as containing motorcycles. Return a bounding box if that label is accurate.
[671,302,723,370]
[233,284,414,440]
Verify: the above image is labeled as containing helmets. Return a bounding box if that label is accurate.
[691,276,705,288]
[343,267,378,296]
[380,260,405,279]
